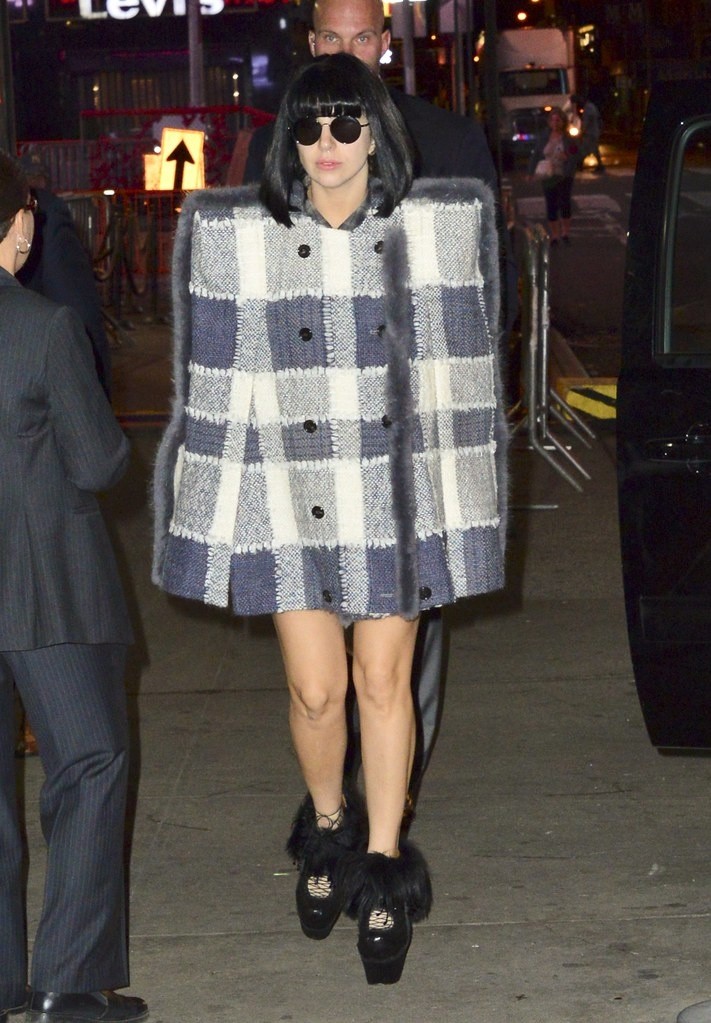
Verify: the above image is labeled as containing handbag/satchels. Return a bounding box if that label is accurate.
[535,159,553,177]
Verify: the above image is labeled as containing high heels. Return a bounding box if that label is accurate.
[287,782,369,940]
[347,844,432,985]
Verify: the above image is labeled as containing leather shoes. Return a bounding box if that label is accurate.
[25,991,149,1023]
[1,984,33,1023]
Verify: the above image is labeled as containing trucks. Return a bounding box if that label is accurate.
[469,22,576,169]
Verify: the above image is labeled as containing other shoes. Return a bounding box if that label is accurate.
[592,165,606,174]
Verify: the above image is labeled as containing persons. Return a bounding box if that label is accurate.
[570,91,606,174]
[244,2,501,847]
[0,148,151,1023]
[151,54,510,989]
[529,110,580,248]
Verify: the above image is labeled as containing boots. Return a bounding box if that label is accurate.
[562,218,570,240]
[548,220,560,244]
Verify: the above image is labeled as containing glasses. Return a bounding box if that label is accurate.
[286,116,371,147]
[22,197,38,212]
